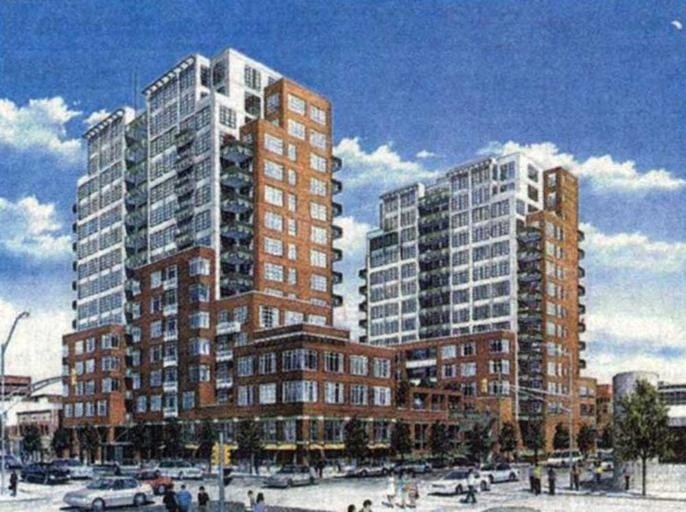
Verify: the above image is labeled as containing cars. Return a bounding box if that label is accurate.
[345,459,521,495]
[267,464,316,488]
[0,453,205,512]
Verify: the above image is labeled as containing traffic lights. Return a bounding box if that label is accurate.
[210,442,219,466]
[48,395,64,403]
[223,444,233,466]
[68,395,89,403]
[223,468,234,485]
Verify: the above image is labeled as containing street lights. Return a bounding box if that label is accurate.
[0,311,33,492]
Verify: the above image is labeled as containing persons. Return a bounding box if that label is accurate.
[9,470,18,497]
[247,490,266,512]
[383,470,421,509]
[529,460,557,496]
[346,499,373,512]
[461,468,477,503]
[158,438,185,462]
[570,452,632,491]
[162,483,210,512]
[111,458,121,475]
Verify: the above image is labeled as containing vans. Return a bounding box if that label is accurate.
[547,449,585,467]
